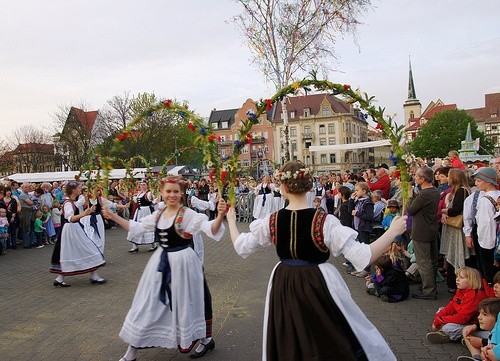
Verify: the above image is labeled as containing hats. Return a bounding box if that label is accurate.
[387,200,398,208]
[471,167,497,185]
[374,164,389,170]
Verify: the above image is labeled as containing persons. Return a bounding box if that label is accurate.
[226,160,408,361]
[0,176,289,255]
[308,151,500,361]
[50,181,107,287]
[102,177,225,361]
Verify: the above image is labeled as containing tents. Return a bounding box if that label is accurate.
[0,164,198,182]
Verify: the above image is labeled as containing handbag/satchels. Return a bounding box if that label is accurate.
[444,187,466,229]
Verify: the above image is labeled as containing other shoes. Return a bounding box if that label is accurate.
[426,330,451,343]
[122,357,136,361]
[53,279,71,287]
[346,268,356,273]
[356,270,370,277]
[342,263,348,266]
[90,278,107,284]
[415,288,438,294]
[149,246,157,251]
[367,288,376,295]
[0,237,58,254]
[457,356,482,361]
[188,338,215,358]
[128,248,138,252]
[412,291,437,300]
[351,271,360,275]
